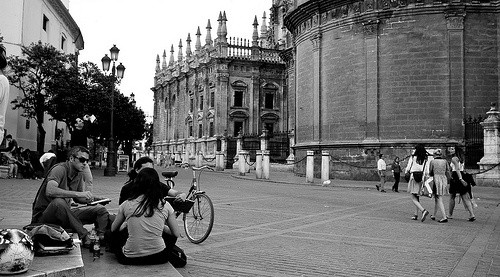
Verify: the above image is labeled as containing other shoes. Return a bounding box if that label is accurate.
[82,236,91,247]
[467,216,476,222]
[421,209,429,222]
[430,215,435,220]
[411,215,418,220]
[98,236,104,245]
[470,204,478,208]
[446,215,453,219]
[438,218,448,223]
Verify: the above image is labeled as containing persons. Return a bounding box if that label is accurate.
[427,149,453,222]
[376,154,387,192]
[107,169,181,265]
[448,146,477,222]
[0,44,9,145]
[159,151,176,168]
[392,156,403,192]
[404,144,429,222]
[119,157,186,208]
[0,135,57,178]
[31,146,110,249]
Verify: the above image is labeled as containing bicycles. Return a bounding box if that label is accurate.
[161,162,217,244]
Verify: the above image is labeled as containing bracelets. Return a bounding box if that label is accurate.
[459,178,462,181]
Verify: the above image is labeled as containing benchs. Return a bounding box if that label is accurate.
[0,225,85,277]
[81,224,184,277]
[0,166,10,178]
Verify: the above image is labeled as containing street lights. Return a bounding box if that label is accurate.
[100,43,127,176]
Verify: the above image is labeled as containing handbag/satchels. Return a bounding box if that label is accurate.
[413,171,423,183]
[27,224,73,257]
[404,170,411,183]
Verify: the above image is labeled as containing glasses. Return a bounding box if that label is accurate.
[73,155,89,163]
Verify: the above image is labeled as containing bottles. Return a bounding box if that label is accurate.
[93,238,100,262]
[89,226,97,253]
